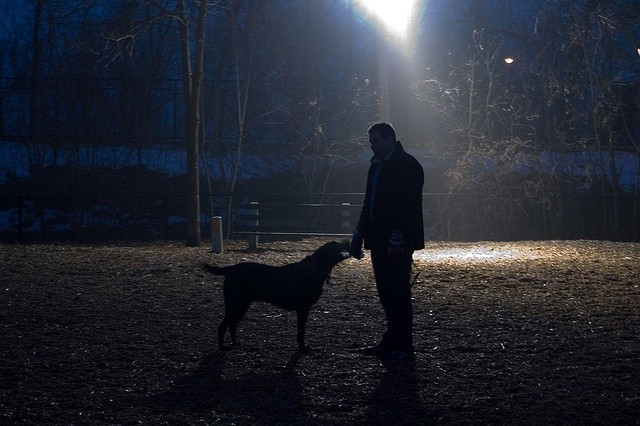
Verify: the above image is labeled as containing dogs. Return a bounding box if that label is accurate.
[202,242,352,354]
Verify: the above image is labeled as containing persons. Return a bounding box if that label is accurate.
[350,123,425,357]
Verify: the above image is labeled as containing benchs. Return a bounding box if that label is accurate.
[231,203,364,250]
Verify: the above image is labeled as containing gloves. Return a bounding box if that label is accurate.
[349,235,365,260]
[379,233,405,264]
